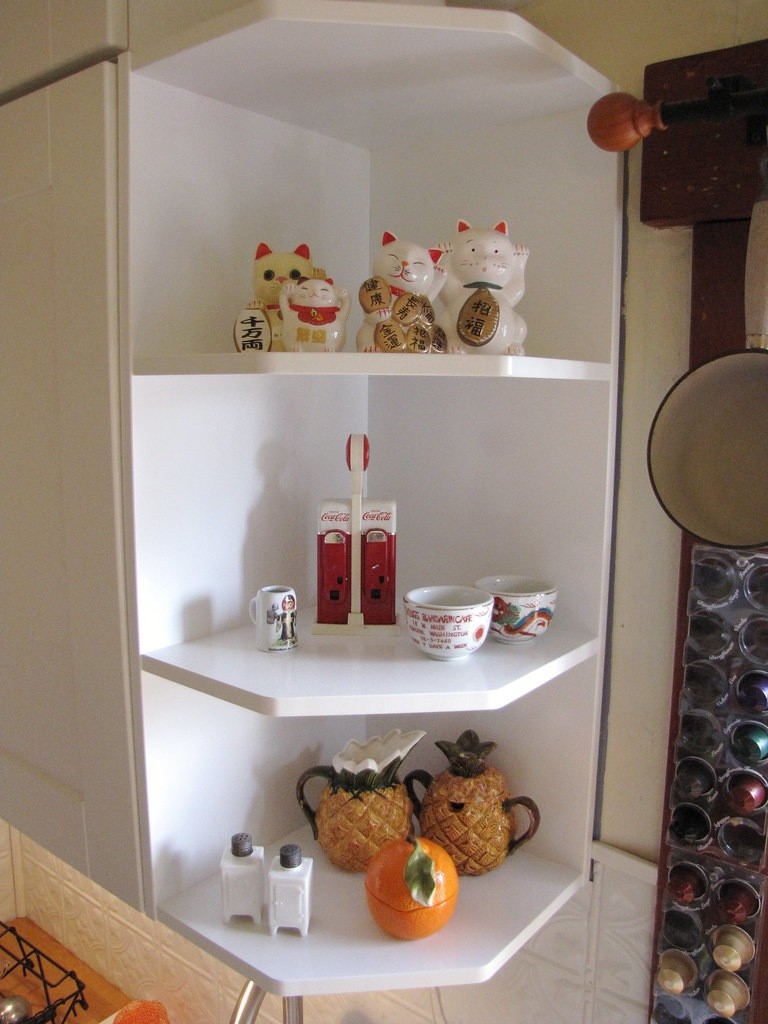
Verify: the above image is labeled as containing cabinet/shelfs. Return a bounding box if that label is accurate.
[121,1,630,1000]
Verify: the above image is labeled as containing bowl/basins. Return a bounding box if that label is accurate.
[648,348,768,550]
[657,674,768,1016]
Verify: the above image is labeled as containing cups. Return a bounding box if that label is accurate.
[472,575,558,645]
[403,586,494,662]
[248,585,299,653]
